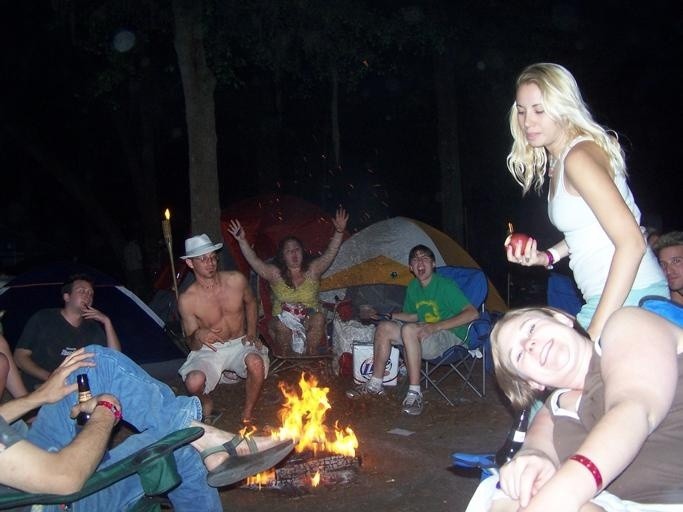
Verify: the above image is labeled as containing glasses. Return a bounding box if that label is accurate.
[187,253,220,261]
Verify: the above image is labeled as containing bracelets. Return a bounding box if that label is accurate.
[568,454,602,499]
[546,247,561,265]
[543,250,554,272]
[335,229,343,234]
[97,401,121,424]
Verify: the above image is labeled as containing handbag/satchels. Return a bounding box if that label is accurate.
[352,341,401,387]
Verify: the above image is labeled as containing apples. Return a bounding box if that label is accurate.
[508,232,532,256]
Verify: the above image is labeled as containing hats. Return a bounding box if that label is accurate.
[179,233,224,260]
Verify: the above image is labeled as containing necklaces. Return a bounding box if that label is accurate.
[197,282,216,289]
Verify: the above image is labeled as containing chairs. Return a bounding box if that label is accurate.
[0,423,204,511]
[256,256,336,387]
[399,263,492,407]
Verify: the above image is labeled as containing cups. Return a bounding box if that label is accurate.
[335,299,353,321]
[360,304,373,320]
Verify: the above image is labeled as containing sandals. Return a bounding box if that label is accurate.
[199,432,297,488]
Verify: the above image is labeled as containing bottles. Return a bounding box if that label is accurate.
[495,407,528,467]
[284,302,306,316]
[75,373,94,436]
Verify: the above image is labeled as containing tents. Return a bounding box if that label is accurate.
[151,192,354,340]
[309,214,510,365]
[0,269,188,362]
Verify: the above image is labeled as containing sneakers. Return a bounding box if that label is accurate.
[399,389,426,417]
[345,383,386,402]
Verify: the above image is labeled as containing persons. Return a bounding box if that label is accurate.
[0,333,36,423]
[501,61,672,438]
[177,232,270,427]
[227,205,349,353]
[649,230,681,304]
[14,275,124,392]
[462,304,681,512]
[345,243,479,417]
[0,343,295,512]
